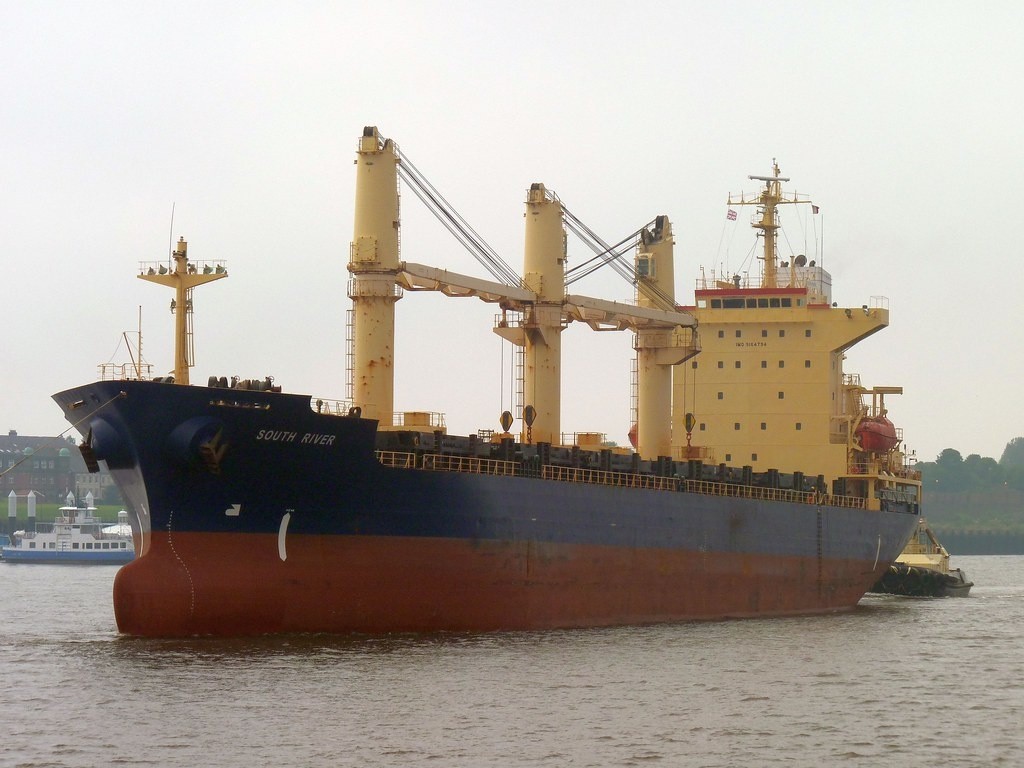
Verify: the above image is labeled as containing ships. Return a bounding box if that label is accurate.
[50,126,923,639]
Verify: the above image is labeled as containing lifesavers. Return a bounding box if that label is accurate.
[808,497,813,504]
[349,406,361,417]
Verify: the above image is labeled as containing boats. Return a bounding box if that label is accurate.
[1,500,136,563]
[866,514,974,599]
[856,413,897,453]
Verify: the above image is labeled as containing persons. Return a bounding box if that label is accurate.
[726,472,738,496]
[679,476,686,492]
[671,474,680,491]
[426,457,433,469]
[806,486,815,504]
[832,302,837,306]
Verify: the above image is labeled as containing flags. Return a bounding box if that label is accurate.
[727,209,737,221]
[812,205,819,214]
